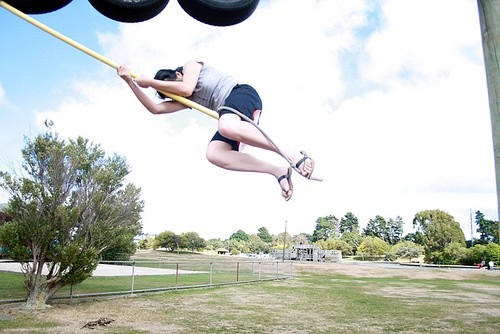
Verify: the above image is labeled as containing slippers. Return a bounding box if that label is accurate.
[290,151,315,179]
[277,168,294,201]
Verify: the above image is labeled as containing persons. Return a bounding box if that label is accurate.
[116,60,316,203]
